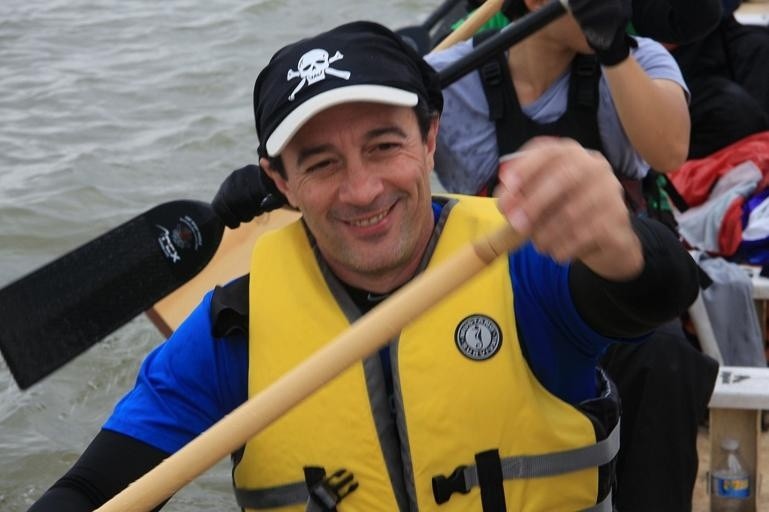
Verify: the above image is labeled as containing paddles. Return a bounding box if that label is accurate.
[144,0,509,340]
[1,1,569,393]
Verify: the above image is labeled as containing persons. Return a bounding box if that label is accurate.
[27,20,697,512]
[418,1,769,512]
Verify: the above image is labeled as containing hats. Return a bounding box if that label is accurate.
[252,21,443,169]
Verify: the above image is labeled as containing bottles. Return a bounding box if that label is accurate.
[709,440,752,511]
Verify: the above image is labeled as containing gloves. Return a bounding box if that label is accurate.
[570,0,634,67]
